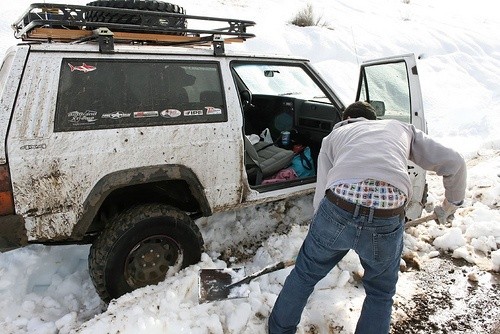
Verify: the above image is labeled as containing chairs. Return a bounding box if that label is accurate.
[201,92,294,181]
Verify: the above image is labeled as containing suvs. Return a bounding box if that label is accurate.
[0,0,428,305]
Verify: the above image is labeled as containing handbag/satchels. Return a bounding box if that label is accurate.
[290,145,316,178]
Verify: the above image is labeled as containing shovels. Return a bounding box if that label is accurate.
[196,212,451,306]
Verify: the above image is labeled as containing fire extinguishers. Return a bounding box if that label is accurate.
[279,129,291,148]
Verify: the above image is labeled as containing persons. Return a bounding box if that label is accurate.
[266,100,467,334]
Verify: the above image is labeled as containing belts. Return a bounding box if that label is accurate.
[325,189,408,219]
